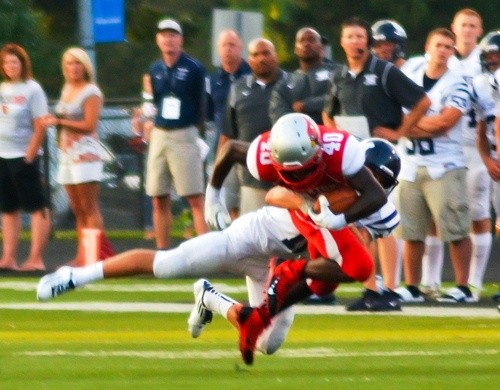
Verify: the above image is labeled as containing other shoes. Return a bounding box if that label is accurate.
[1,265,44,278]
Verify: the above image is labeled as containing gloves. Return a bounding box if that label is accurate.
[308,198,348,231]
[204,184,232,231]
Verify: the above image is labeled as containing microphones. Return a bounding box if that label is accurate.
[358,49,364,54]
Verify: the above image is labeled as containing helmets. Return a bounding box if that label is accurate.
[370,20,408,42]
[479,30,500,55]
[269,112,321,168]
[350,137,401,193]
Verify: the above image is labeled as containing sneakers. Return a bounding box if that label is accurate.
[393,285,427,305]
[437,286,481,304]
[36,265,78,301]
[347,289,401,312]
[237,305,271,365]
[267,257,308,316]
[188,278,214,338]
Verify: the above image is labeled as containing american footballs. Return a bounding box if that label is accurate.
[313,187,361,216]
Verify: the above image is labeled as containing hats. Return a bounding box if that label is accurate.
[156,19,183,36]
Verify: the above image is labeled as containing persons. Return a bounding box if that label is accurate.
[39,46,106,269]
[0,43,52,276]
[35,136,405,349]
[141,18,210,252]
[131,8,499,309]
[203,110,386,360]
